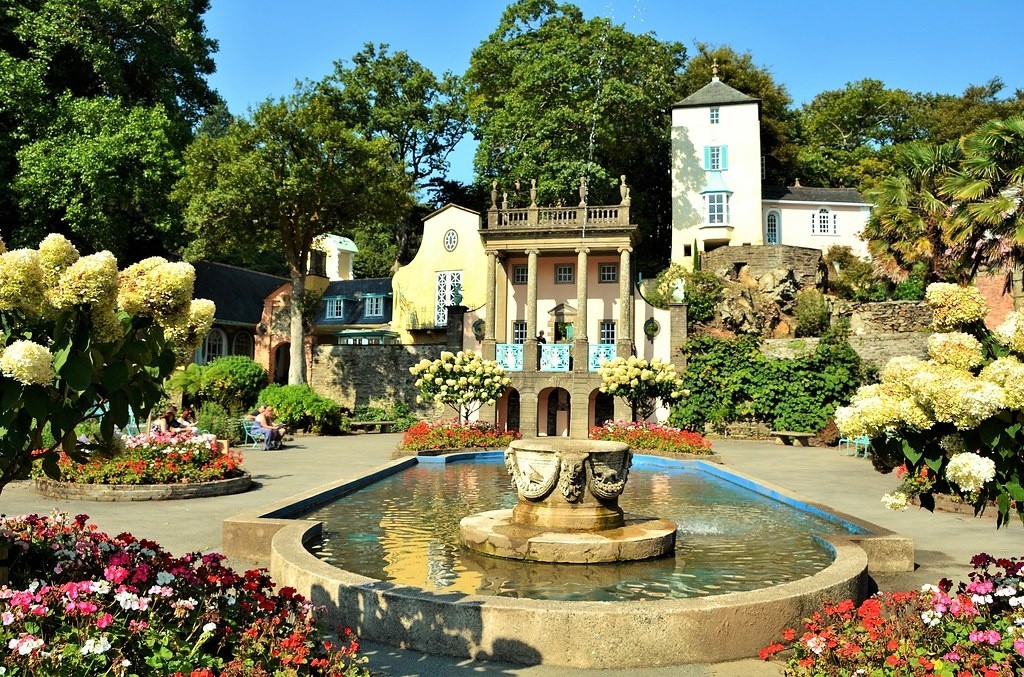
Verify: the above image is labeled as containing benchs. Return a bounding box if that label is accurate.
[351,421,397,433]
[770,431,816,447]
[241,419,266,450]
[839,433,870,459]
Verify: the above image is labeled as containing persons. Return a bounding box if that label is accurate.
[150,407,198,437]
[245,405,286,451]
[536,331,546,371]
